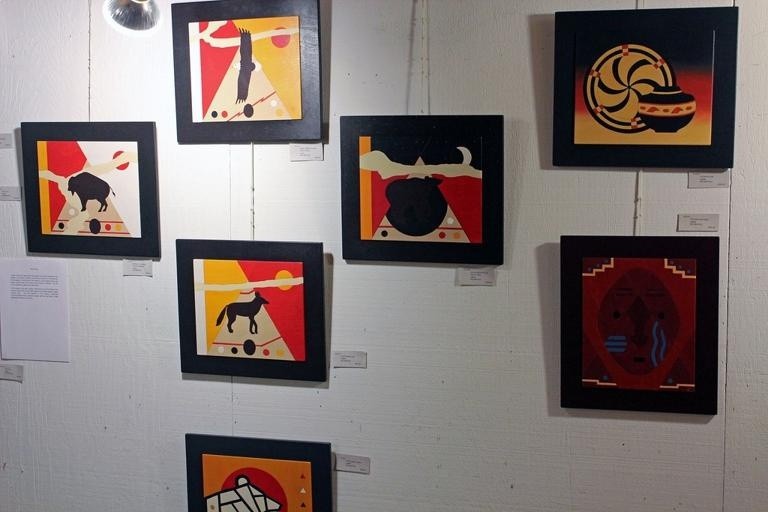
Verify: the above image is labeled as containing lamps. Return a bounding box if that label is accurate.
[100,0,164,40]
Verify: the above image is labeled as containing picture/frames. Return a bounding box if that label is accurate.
[339,113,506,263]
[169,1,327,143]
[173,236,331,384]
[557,233,722,417]
[550,8,738,169]
[181,431,333,512]
[15,120,164,261]
